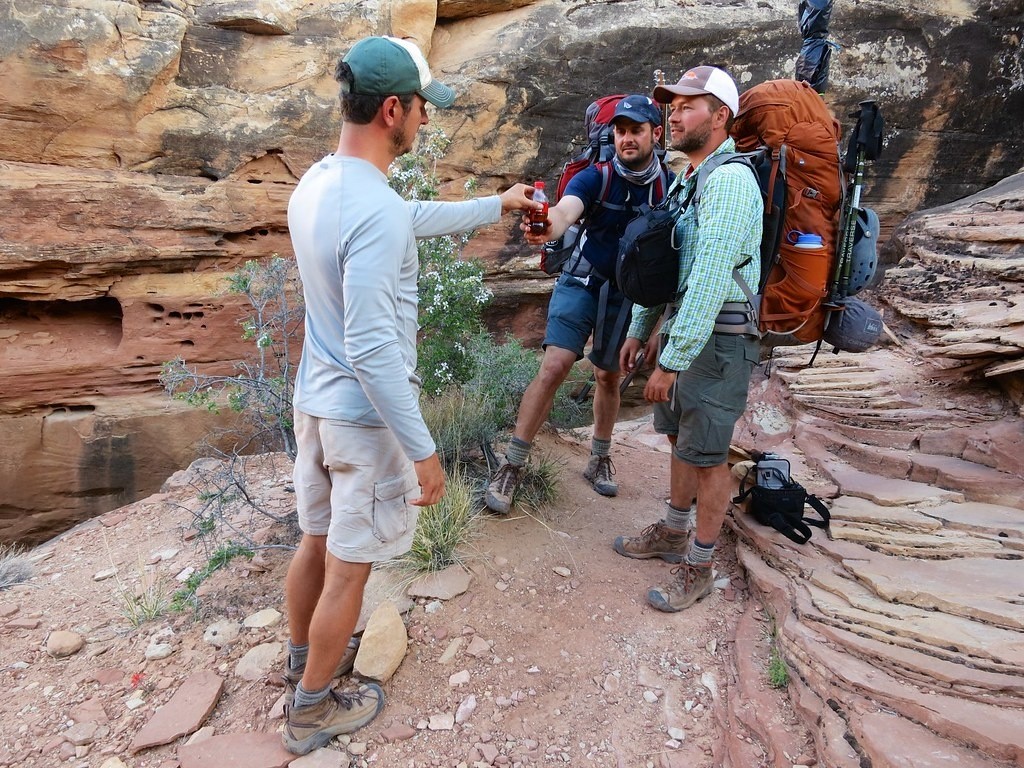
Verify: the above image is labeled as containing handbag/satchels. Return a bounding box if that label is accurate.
[822,296,883,353]
[616,203,679,307]
[732,465,830,545]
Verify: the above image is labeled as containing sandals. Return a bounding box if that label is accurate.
[848,207,880,295]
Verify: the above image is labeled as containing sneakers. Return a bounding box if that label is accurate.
[615,519,692,563]
[281,637,359,701]
[583,452,618,496]
[649,554,715,612]
[281,682,384,756]
[485,455,525,514]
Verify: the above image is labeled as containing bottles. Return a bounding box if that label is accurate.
[787,230,823,249]
[527,182,549,235]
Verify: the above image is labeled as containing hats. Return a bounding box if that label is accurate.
[339,35,456,108]
[608,93,662,124]
[653,66,739,119]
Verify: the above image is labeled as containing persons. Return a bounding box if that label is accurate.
[281,33,543,754]
[483,95,679,514]
[613,64,764,612]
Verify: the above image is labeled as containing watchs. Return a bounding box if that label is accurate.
[658,362,681,374]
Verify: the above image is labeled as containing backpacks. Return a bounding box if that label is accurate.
[695,80,843,344]
[541,94,668,275]
